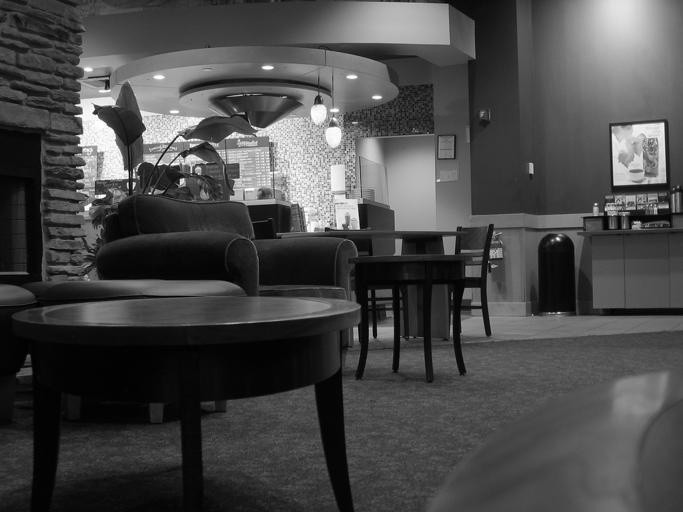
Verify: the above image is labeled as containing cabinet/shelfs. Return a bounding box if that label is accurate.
[574,227,683,315]
[334,201,394,256]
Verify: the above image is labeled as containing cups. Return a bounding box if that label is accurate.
[607,215,618,230]
[619,215,631,230]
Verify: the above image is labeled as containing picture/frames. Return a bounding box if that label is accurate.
[436,134,456,160]
[608,119,672,192]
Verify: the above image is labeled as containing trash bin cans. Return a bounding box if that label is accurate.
[537,232,576,317]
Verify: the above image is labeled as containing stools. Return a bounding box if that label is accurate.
[1,276,249,425]
[349,254,472,384]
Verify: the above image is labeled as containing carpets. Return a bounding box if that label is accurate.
[1,330,681,509]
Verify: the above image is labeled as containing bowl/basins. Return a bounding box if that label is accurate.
[629,169,644,182]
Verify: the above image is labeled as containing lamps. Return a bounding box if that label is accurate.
[309,68,342,149]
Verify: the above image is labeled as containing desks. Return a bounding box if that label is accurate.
[272,230,471,341]
[8,294,372,509]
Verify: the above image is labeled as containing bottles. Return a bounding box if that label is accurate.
[670,184,683,214]
[592,202,599,217]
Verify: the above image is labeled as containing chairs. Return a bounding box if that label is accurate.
[447,224,494,336]
[94,192,355,298]
